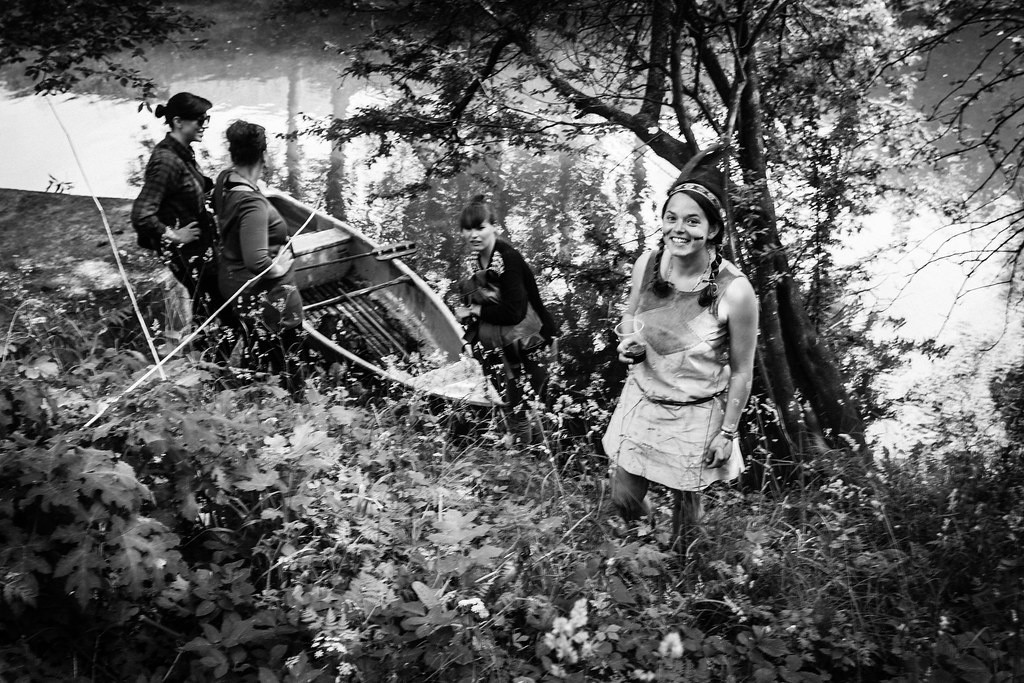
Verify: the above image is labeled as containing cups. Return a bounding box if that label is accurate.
[614,319,646,365]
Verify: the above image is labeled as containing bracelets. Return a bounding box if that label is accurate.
[720,426,742,441]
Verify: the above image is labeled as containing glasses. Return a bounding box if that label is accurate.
[194,115,212,127]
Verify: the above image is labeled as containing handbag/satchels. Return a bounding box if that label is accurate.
[468,266,545,379]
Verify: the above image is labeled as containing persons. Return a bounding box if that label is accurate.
[452,194,556,457]
[208,119,310,406]
[601,168,759,556]
[131,91,219,368]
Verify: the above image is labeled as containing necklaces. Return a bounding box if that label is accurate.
[666,246,712,293]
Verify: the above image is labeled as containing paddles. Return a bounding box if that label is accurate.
[294,240,418,272]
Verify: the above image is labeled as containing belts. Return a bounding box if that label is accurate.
[632,377,726,405]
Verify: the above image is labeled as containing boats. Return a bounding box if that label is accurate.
[254,178,507,428]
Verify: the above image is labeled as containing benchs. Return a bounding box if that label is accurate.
[289,228,353,290]
